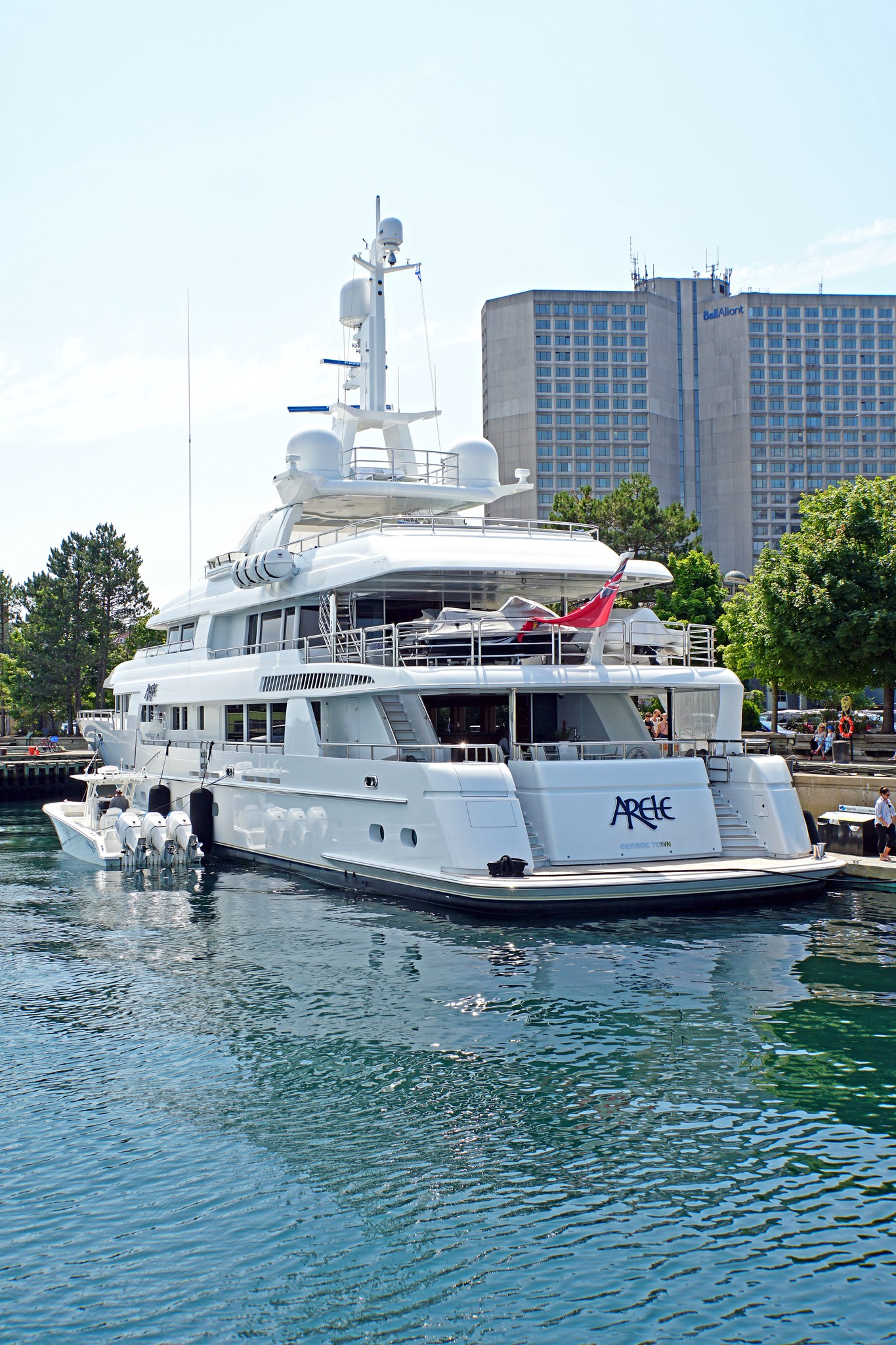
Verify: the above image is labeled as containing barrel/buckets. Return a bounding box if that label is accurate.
[0,747,8,756]
[88,744,93,750]
[28,747,36,755]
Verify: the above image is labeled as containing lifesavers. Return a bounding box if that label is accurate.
[839,716,854,738]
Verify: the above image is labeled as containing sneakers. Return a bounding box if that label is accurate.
[812,750,818,754]
[822,756,826,761]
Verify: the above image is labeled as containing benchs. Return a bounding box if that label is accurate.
[784,744,832,750]
[860,746,896,757]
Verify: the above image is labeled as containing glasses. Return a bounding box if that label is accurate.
[820,727,825,729]
[884,793,890,797]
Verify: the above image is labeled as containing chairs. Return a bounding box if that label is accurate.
[490,746,510,766]
[557,746,581,759]
[100,807,142,837]
[529,746,549,761]
[514,747,525,761]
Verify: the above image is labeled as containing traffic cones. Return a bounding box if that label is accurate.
[35,745,40,755]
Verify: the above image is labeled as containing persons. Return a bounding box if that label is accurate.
[447,718,459,732]
[642,710,679,751]
[498,729,510,755]
[874,786,896,861]
[108,787,130,813]
[808,723,836,761]
[888,750,896,765]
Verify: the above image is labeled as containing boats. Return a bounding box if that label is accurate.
[77,192,847,915]
[42,764,205,867]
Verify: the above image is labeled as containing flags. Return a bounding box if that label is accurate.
[517,558,630,643]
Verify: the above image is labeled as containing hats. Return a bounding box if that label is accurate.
[826,726,834,731]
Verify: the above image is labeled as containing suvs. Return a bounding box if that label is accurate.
[758,712,840,734]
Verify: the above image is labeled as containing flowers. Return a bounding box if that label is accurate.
[553,720,575,741]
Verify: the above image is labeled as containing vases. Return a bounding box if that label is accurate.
[559,741,570,747]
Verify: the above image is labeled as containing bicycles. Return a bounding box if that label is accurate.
[37,734,60,753]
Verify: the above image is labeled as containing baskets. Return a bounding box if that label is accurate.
[49,736,58,742]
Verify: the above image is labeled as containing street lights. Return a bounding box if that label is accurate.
[723,570,779,738]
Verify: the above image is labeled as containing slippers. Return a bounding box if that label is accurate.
[884,856,891,860]
[808,758,812,761]
[810,751,813,753]
[894,763,896,765]
[888,758,896,761]
[879,858,888,861]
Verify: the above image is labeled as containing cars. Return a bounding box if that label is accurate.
[763,708,896,733]
[61,722,77,735]
[755,720,804,734]
